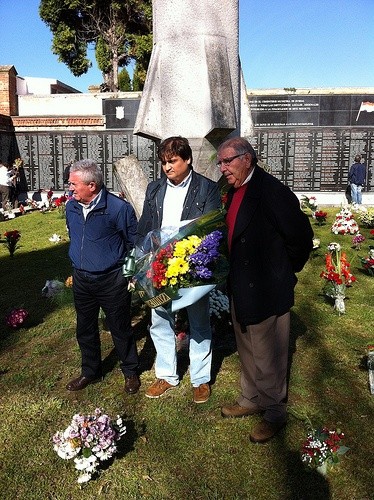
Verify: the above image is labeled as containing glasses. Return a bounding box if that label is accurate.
[216,154,245,168]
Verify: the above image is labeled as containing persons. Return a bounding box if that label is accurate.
[0,162,17,211]
[217,136,314,443]
[348,155,365,204]
[135,137,221,403]
[64,159,78,196]
[66,158,140,394]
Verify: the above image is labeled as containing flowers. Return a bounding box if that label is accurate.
[123,186,232,352]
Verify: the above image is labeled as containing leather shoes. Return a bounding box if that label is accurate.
[66,375,101,392]
[124,374,141,394]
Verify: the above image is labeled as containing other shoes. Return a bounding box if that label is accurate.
[144,379,179,399]
[221,402,262,417]
[250,420,287,444]
[193,383,210,404]
